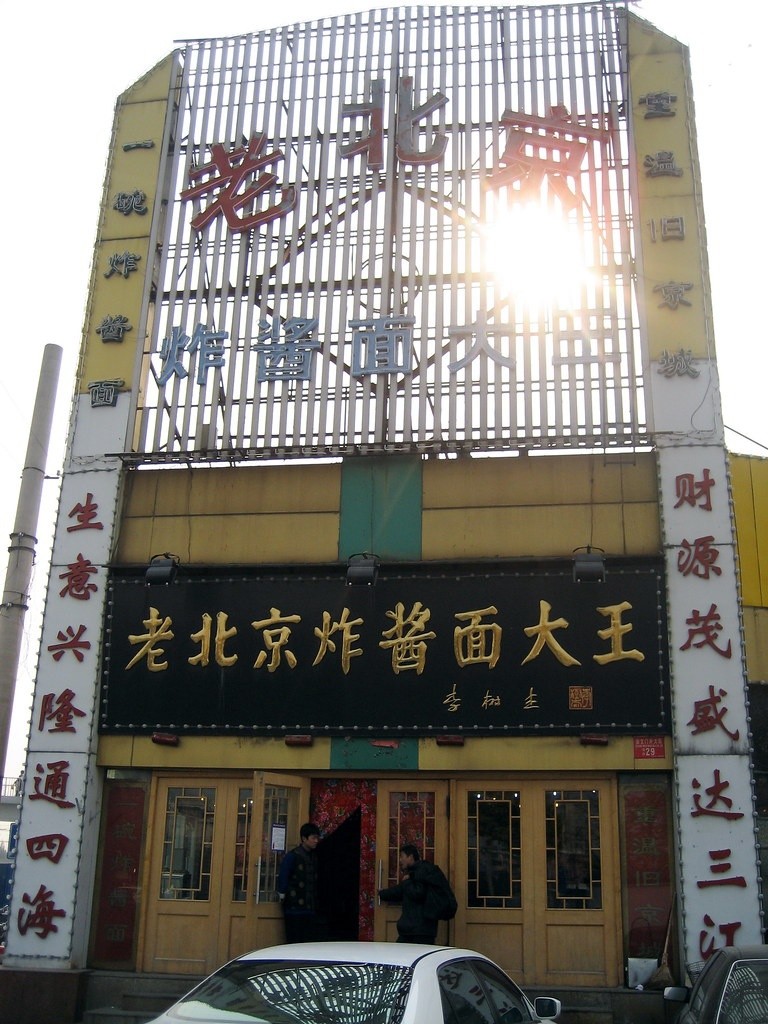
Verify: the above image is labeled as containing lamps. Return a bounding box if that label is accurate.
[580,735,609,748]
[153,732,181,749]
[345,551,384,590]
[435,735,465,748]
[284,734,313,748]
[143,552,181,588]
[571,544,608,585]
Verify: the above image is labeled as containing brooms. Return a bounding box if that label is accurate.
[641,891,678,990]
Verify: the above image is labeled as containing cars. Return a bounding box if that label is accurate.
[663,946,768,1024]
[142,942,563,1024]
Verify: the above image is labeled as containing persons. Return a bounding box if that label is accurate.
[379,844,437,944]
[275,823,325,945]
